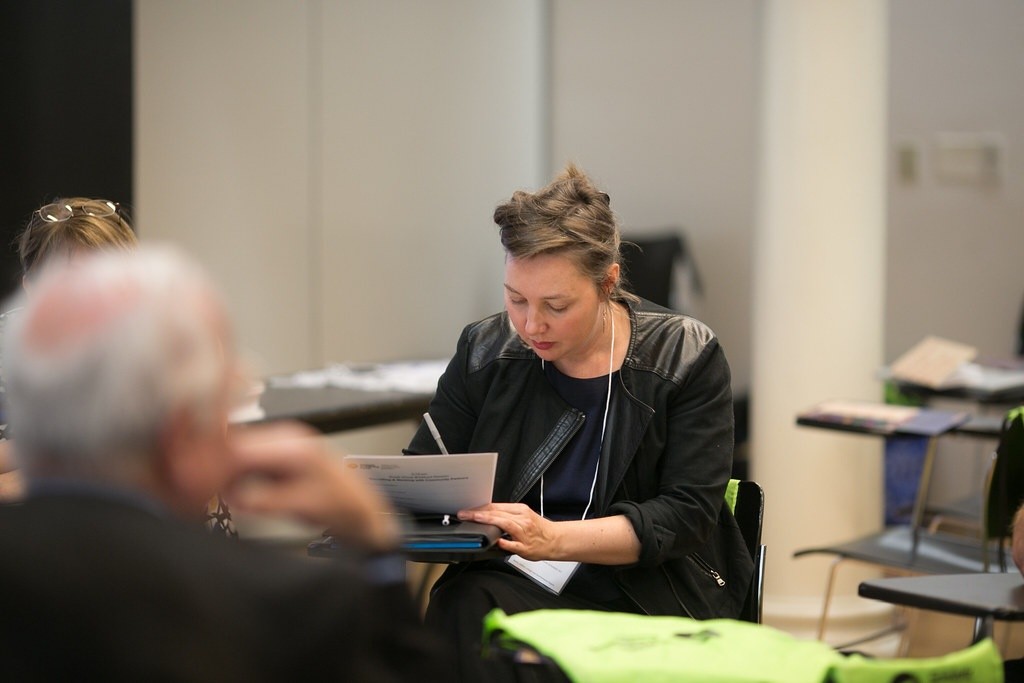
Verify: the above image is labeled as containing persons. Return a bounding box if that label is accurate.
[0,193,561,683]
[398,160,735,683]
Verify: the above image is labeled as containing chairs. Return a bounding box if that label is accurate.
[790,402,1024,660]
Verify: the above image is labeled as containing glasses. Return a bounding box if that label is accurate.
[29,199,121,240]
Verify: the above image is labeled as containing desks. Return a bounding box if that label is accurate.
[220,355,458,453]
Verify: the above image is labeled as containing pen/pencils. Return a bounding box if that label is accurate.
[441,512,451,525]
[423,412,448,455]
